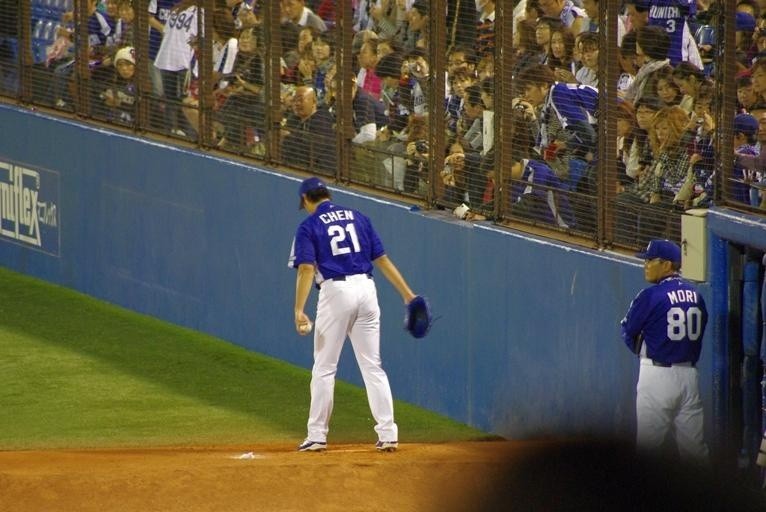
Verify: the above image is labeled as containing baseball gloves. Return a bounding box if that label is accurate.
[404,295,432,339]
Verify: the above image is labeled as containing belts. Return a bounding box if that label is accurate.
[333,273,372,281]
[652,359,697,367]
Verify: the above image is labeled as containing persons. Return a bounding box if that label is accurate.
[287,178,418,452]
[2,1,765,269]
[621,239,709,509]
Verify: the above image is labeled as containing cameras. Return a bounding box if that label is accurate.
[514,103,526,113]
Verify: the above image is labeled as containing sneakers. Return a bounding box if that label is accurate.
[376,441,398,449]
[299,440,327,451]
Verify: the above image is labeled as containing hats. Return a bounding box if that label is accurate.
[636,239,681,260]
[736,13,756,31]
[114,46,136,68]
[735,114,759,132]
[299,178,325,209]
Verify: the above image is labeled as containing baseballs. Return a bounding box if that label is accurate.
[299,322,313,334]
